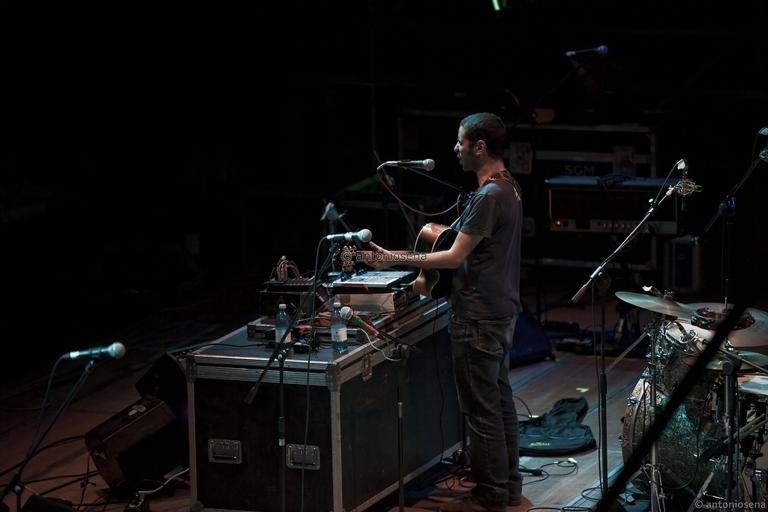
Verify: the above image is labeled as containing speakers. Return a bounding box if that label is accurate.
[135,342,209,423]
[84,395,189,501]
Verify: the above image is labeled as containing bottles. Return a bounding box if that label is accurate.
[330,302,348,355]
[276,303,293,359]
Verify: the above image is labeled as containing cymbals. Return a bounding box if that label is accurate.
[683,348,767,371]
[615,292,698,318]
[664,303,768,347]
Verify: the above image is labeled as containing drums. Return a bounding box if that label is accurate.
[648,316,743,403]
[618,378,768,497]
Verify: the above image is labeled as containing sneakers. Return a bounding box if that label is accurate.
[439,492,522,512]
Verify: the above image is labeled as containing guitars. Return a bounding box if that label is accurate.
[333,222,457,300]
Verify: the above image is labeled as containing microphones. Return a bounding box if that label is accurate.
[70,342,126,360]
[339,306,385,341]
[674,160,702,196]
[327,228,372,243]
[565,45,609,57]
[679,323,700,354]
[386,158,435,171]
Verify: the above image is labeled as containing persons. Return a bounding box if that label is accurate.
[355,112,525,511]
